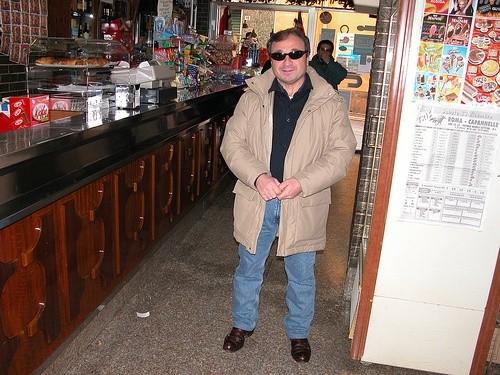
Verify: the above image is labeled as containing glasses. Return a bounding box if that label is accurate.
[271,50,308,61]
[320,47,332,52]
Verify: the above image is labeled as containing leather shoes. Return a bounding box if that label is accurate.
[224,327,254,352]
[290,339,312,362]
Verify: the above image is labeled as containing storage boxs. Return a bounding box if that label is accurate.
[8,93,50,129]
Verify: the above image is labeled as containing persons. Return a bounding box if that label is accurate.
[308,40,347,92]
[219,28,357,364]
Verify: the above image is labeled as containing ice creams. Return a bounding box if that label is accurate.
[430,87,436,100]
[442,55,464,73]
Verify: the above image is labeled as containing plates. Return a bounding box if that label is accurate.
[467,22,500,106]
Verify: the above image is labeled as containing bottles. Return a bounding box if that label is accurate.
[78,22,88,38]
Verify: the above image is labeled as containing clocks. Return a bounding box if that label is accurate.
[320,11,332,24]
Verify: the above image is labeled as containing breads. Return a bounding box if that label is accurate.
[36,57,112,65]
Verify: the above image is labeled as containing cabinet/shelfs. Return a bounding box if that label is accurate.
[26,37,130,113]
